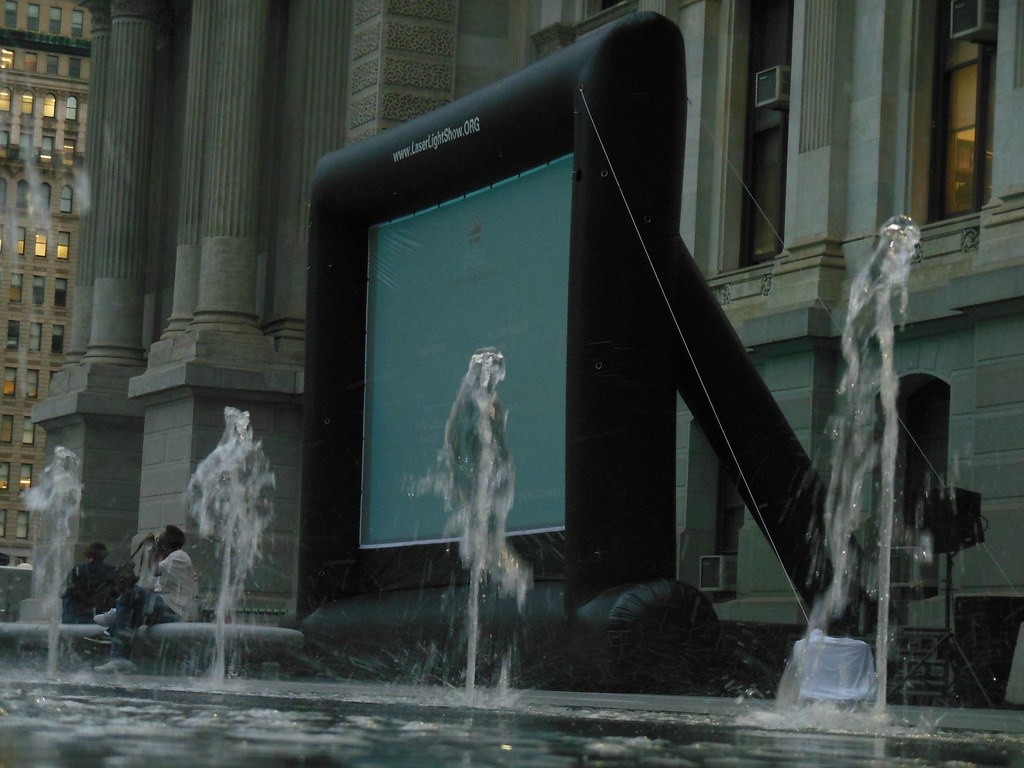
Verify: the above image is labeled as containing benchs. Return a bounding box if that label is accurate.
[0,620,305,682]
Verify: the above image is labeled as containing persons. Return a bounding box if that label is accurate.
[93,525,199,674]
[58,541,118,624]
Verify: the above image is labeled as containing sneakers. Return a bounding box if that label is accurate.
[93,658,133,674]
[93,607,129,628]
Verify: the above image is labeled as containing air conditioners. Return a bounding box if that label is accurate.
[889,546,939,588]
[756,65,790,108]
[933,0,999,41]
[697,554,737,593]
